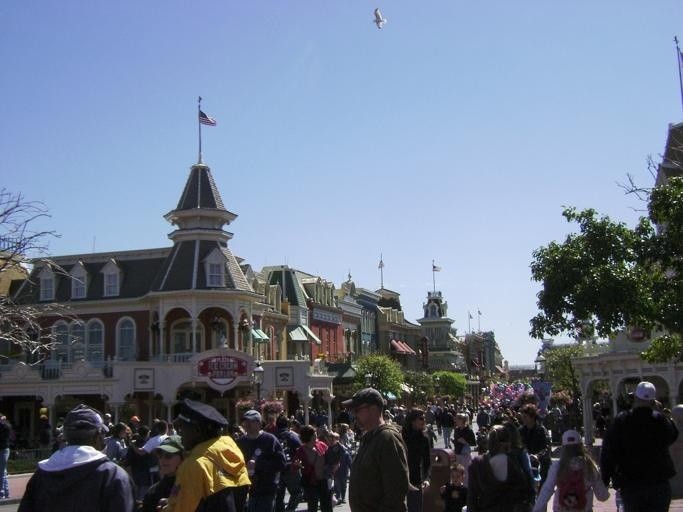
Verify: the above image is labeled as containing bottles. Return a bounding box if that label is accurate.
[173,396,229,436]
[62,404,109,444]
[240,409,261,425]
[340,387,382,407]
[635,381,656,400]
[562,429,582,446]
[153,435,185,456]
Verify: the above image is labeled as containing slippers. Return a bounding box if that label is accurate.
[557,468,587,509]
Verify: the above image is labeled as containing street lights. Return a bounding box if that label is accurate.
[299,468,314,487]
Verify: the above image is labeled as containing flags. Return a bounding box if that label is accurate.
[199,110,216,126]
[433,264,442,272]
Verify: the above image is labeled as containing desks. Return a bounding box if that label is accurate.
[434,377,440,396]
[253,361,265,406]
[365,371,371,387]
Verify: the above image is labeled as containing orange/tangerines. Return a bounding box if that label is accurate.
[528,455,542,497]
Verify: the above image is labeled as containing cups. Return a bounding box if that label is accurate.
[350,404,371,414]
[417,416,426,420]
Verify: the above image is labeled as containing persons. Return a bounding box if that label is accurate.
[1,387,633,512]
[600,381,679,512]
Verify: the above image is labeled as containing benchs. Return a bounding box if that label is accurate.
[433,264,442,272]
[199,110,216,126]
